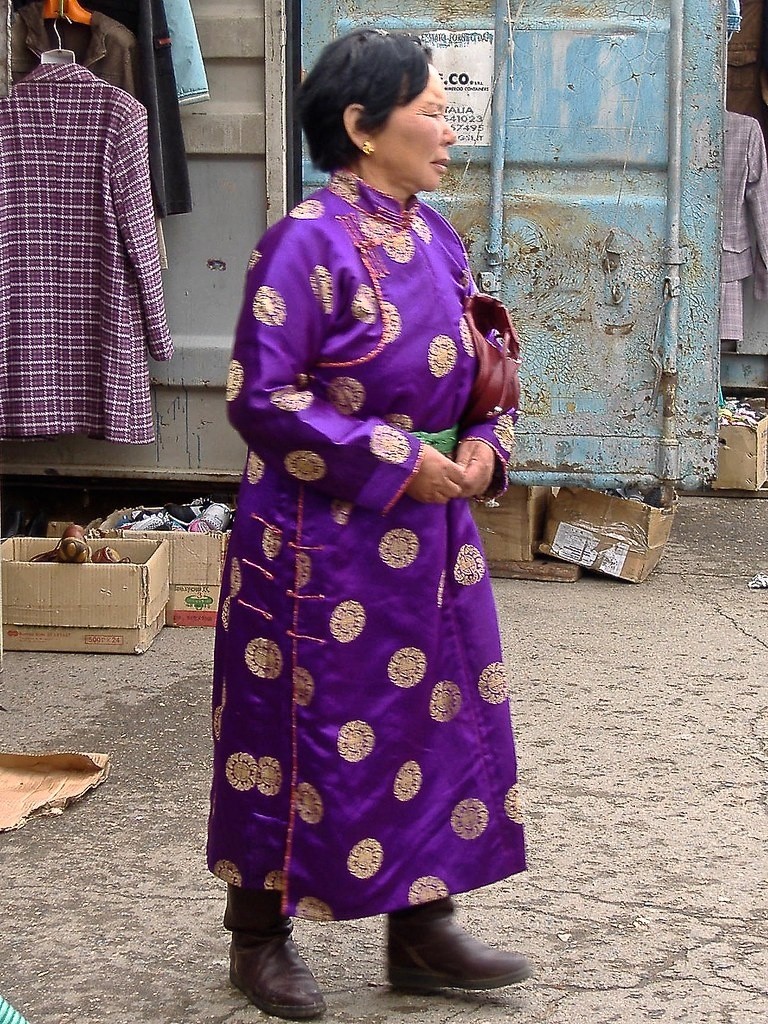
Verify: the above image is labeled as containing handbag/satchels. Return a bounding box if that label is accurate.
[460,294,521,432]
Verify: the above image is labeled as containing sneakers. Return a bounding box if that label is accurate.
[114,497,234,532]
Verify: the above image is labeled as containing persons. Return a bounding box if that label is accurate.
[208,28,530,1017]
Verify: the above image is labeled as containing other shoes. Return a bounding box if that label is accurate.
[29,524,91,564]
[92,546,133,565]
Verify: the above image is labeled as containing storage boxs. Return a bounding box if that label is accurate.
[469,487,551,561]
[539,488,678,583]
[84,506,229,627]
[1,536,169,654]
[710,399,768,492]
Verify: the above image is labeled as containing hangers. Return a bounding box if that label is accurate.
[40,0,91,68]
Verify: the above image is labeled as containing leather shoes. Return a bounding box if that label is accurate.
[387,898,529,995]
[228,920,326,1020]
